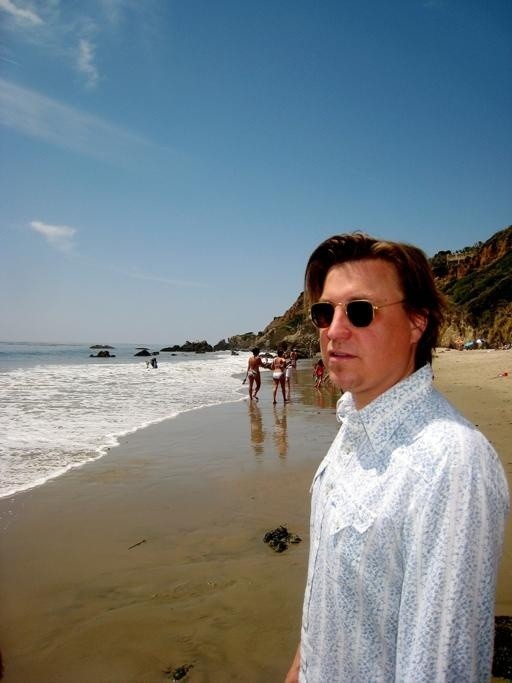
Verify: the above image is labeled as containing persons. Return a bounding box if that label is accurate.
[271,403,288,459]
[312,387,337,407]
[313,357,323,387]
[241,348,298,404]
[249,401,264,444]
[284,232,512,681]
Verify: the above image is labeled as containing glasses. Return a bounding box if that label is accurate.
[307,296,412,330]
